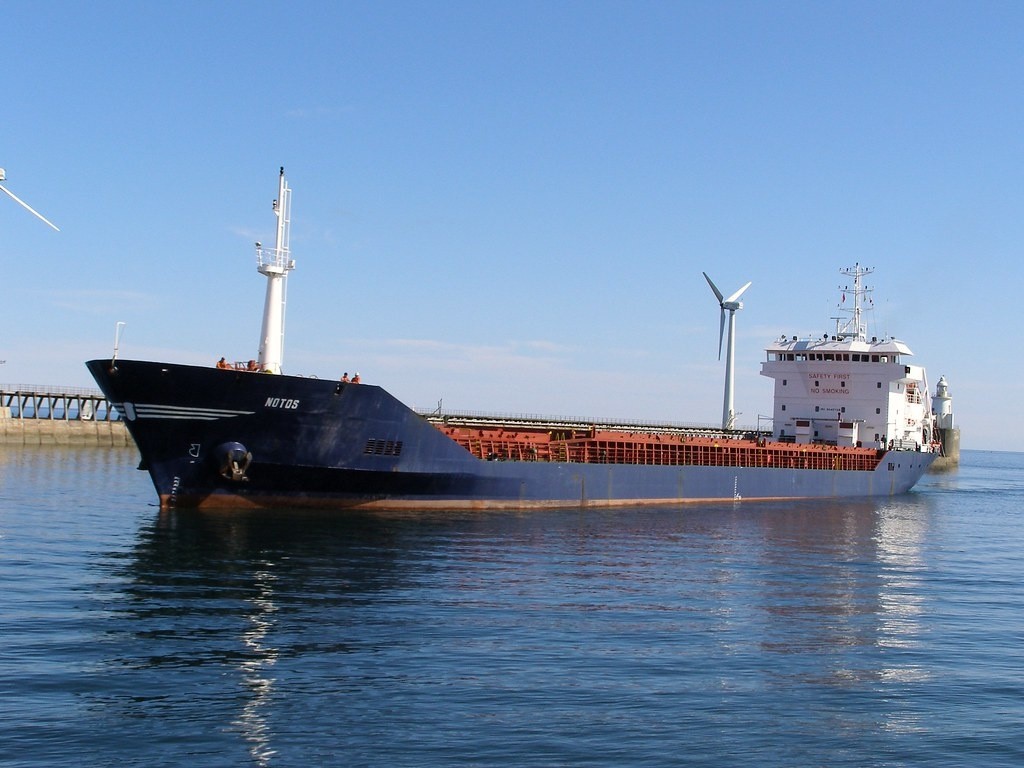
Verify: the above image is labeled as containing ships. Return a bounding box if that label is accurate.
[84,261,941,513]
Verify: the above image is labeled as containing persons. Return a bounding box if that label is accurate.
[888,440,892,451]
[881,434,887,451]
[341,372,350,383]
[351,372,361,384]
[215,357,226,369]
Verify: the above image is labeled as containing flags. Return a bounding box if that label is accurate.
[863,293,867,302]
[842,292,846,302]
[869,294,873,303]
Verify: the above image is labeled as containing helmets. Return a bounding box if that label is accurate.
[355,372,359,376]
